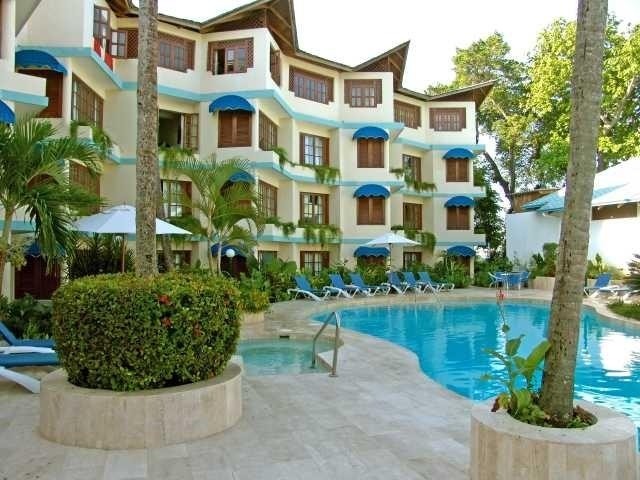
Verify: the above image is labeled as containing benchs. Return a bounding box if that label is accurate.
[584,273,612,298]
[347,274,390,296]
[403,272,439,296]
[381,273,411,296]
[418,272,455,295]
[288,276,330,302]
[488,272,531,290]
[0,353,58,392]
[0,321,56,353]
[323,274,360,298]
[600,287,636,304]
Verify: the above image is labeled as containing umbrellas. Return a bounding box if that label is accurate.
[65,204,192,272]
